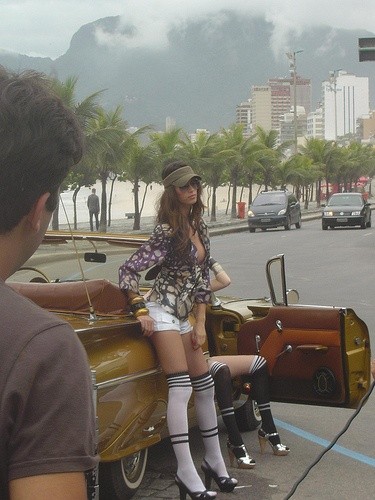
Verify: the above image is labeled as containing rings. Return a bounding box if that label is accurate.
[140,320,142,322]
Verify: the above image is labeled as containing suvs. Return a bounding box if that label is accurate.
[247,188,302,232]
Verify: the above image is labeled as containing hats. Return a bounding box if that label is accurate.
[162,165,202,189]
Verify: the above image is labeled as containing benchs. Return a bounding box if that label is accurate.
[125,213,135,219]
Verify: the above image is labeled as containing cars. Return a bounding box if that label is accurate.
[321,192,371,230]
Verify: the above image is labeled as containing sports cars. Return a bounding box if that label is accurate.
[6,253,375,498]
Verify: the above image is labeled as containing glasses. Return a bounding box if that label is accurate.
[179,179,200,193]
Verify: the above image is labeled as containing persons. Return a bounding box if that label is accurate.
[87,188,100,233]
[188,256,291,469]
[0,64,101,500]
[118,162,237,500]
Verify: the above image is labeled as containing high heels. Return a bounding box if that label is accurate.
[201,457,238,492]
[226,438,256,469]
[257,428,290,456]
[175,473,216,500]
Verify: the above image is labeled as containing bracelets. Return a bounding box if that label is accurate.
[206,257,224,275]
[128,296,149,319]
